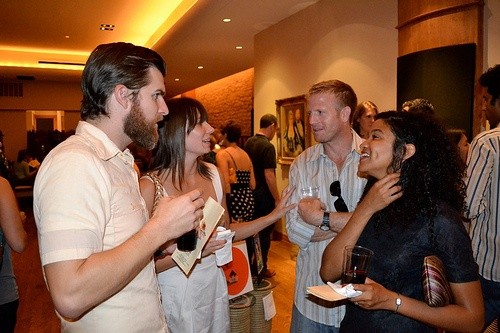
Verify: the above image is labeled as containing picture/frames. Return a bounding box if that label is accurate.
[274,94,313,166]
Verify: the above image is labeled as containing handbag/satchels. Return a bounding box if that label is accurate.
[422,255,454,305]
[228,168,238,184]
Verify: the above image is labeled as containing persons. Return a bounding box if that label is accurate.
[33,42,205,333]
[14,150,37,187]
[283,65,500,333]
[242,113,281,277]
[139,97,297,333]
[255,242,263,272]
[0,176,29,333]
[216,120,255,273]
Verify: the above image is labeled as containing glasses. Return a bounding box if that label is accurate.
[329,181,348,213]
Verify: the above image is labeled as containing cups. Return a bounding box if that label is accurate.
[301,186,320,199]
[341,245,372,285]
[176,225,197,251]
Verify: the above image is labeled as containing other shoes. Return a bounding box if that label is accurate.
[264,269,276,278]
